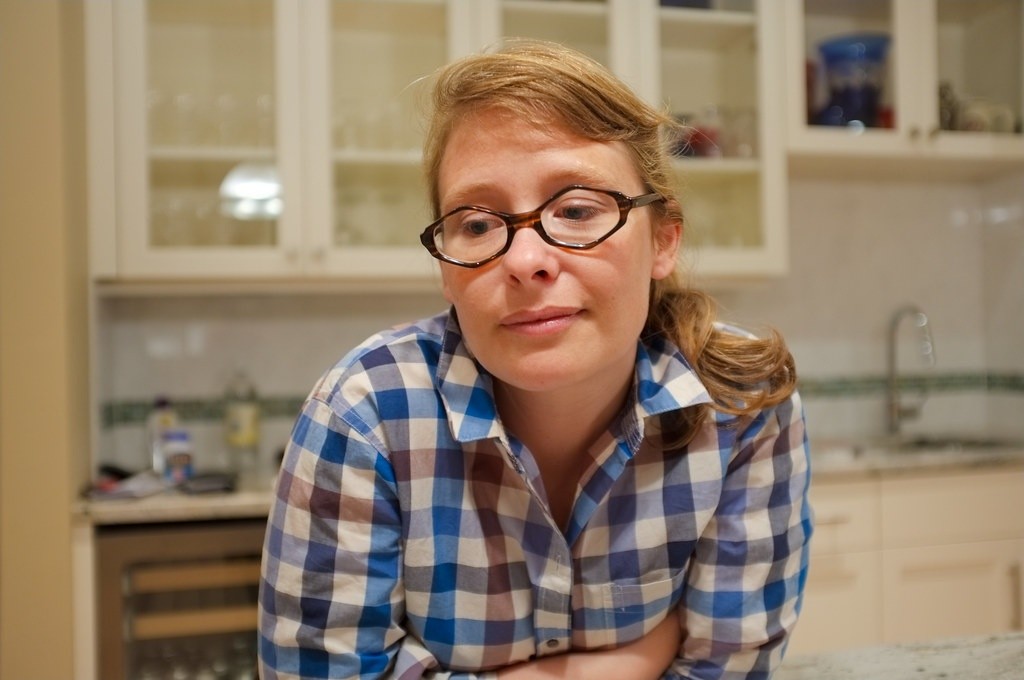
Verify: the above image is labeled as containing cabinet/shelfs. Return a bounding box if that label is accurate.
[84,0,1024,675]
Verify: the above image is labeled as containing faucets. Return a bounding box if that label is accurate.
[883,303,937,446]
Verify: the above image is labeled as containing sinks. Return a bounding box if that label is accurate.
[879,432,1023,458]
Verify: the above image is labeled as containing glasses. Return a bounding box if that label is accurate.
[419,184,660,270]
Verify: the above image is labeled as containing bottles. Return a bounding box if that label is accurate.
[163,429,191,483]
[148,395,178,474]
[219,363,261,490]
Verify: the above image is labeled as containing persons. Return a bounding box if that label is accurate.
[256,37,813,679]
